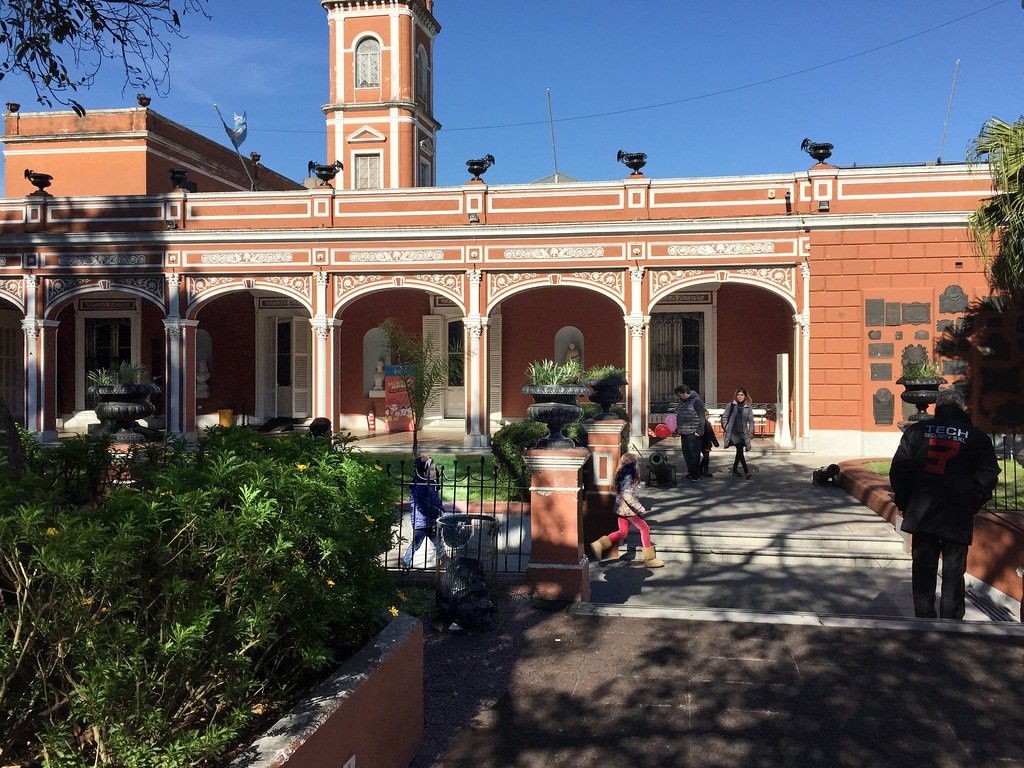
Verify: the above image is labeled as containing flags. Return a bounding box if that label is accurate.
[224,112,247,149]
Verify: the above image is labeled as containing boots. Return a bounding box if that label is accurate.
[591,535,612,561]
[643,546,664,568]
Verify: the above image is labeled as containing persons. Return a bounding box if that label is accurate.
[674,384,720,483]
[398,456,453,570]
[564,342,580,364]
[721,386,755,479]
[889,389,1002,620]
[589,452,664,567]
[196,358,210,390]
[309,417,332,440]
[372,361,388,390]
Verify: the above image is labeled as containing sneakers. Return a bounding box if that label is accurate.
[437,556,450,567]
[400,559,409,570]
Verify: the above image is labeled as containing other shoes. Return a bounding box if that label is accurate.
[703,472,713,477]
[745,473,752,480]
[732,471,743,477]
[681,474,702,481]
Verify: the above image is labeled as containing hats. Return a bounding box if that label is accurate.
[415,455,432,478]
[616,453,636,472]
[935,389,964,409]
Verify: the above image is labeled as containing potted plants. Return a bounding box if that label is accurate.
[86,359,161,443]
[895,359,948,421]
[584,362,628,420]
[520,358,595,449]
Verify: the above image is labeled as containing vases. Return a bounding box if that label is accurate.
[170,169,188,188]
[810,143,834,165]
[626,152,647,175]
[30,173,54,196]
[315,165,338,186]
[466,159,488,180]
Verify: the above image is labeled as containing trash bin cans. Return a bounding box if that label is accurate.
[435,513,500,630]
